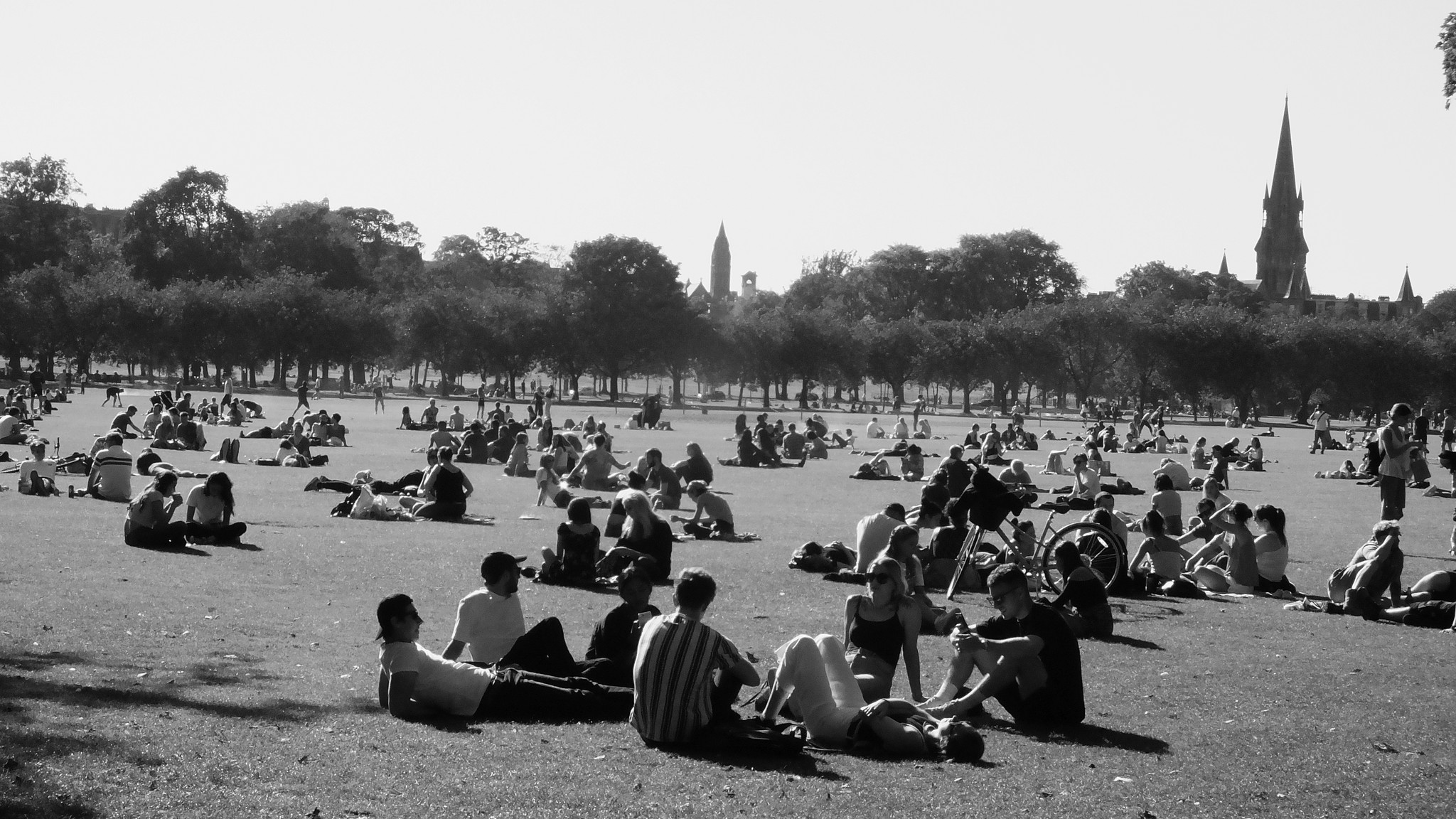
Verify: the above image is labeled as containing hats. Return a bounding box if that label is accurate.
[885,502,909,525]
[482,552,527,582]
[376,593,413,642]
[1094,491,1111,507]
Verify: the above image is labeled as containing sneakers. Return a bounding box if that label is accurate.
[914,695,963,718]
[935,607,963,637]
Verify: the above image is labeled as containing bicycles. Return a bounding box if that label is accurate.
[0,438,81,474]
[946,457,1129,600]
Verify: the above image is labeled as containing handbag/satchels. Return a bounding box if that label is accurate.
[1412,453,1432,483]
[352,483,416,522]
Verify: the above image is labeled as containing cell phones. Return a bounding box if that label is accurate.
[955,613,971,635]
[638,611,651,628]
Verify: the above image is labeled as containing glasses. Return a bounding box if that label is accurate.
[1197,507,1212,519]
[866,572,898,586]
[986,586,1019,605]
[949,720,973,739]
[1074,460,1085,465]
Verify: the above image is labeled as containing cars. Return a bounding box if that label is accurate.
[703,391,725,400]
[578,387,597,395]
[435,381,465,392]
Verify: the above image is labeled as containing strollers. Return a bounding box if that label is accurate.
[154,390,177,410]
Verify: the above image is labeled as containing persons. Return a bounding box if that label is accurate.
[0,362,1456,640]
[1327,519,1405,615]
[292,381,310,416]
[102,387,124,407]
[19,440,59,497]
[584,566,662,689]
[185,471,247,545]
[477,384,485,419]
[78,432,132,503]
[124,470,187,549]
[414,446,474,521]
[755,556,927,721]
[441,551,615,686]
[628,567,760,748]
[1378,403,1424,520]
[670,479,735,539]
[373,382,385,414]
[915,563,1087,732]
[756,634,985,764]
[376,594,634,721]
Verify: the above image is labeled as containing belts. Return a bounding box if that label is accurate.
[845,718,861,753]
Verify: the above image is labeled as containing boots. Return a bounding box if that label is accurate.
[1354,585,1384,618]
[1322,600,1346,614]
[1342,588,1363,617]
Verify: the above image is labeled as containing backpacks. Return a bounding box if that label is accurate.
[1366,426,1392,476]
[310,455,329,466]
[330,485,362,516]
[1160,578,1198,597]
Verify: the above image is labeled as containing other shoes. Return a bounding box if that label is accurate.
[754,666,789,713]
[303,475,327,491]
[798,457,806,467]
[29,470,50,497]
[296,456,308,467]
[953,611,971,635]
[1422,484,1438,497]
[739,715,777,735]
